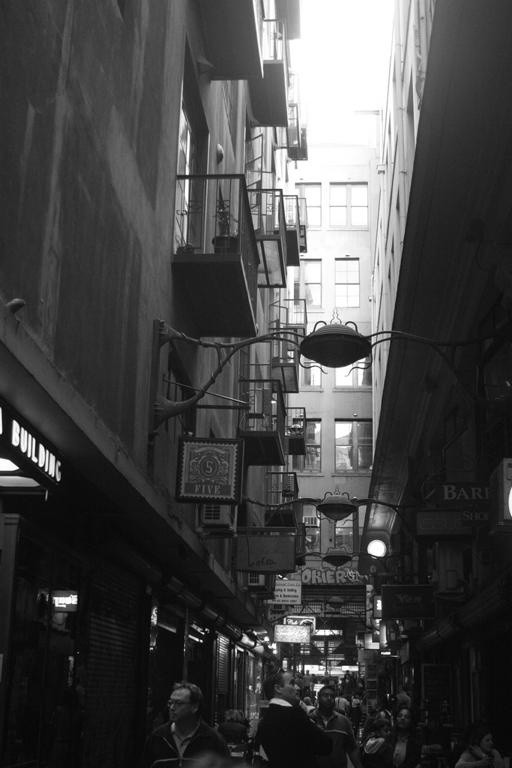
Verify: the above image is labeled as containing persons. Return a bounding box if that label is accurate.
[219,670,503,768]
[143,681,232,768]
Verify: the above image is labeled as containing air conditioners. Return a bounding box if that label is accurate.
[248,574,266,588]
[199,501,231,528]
